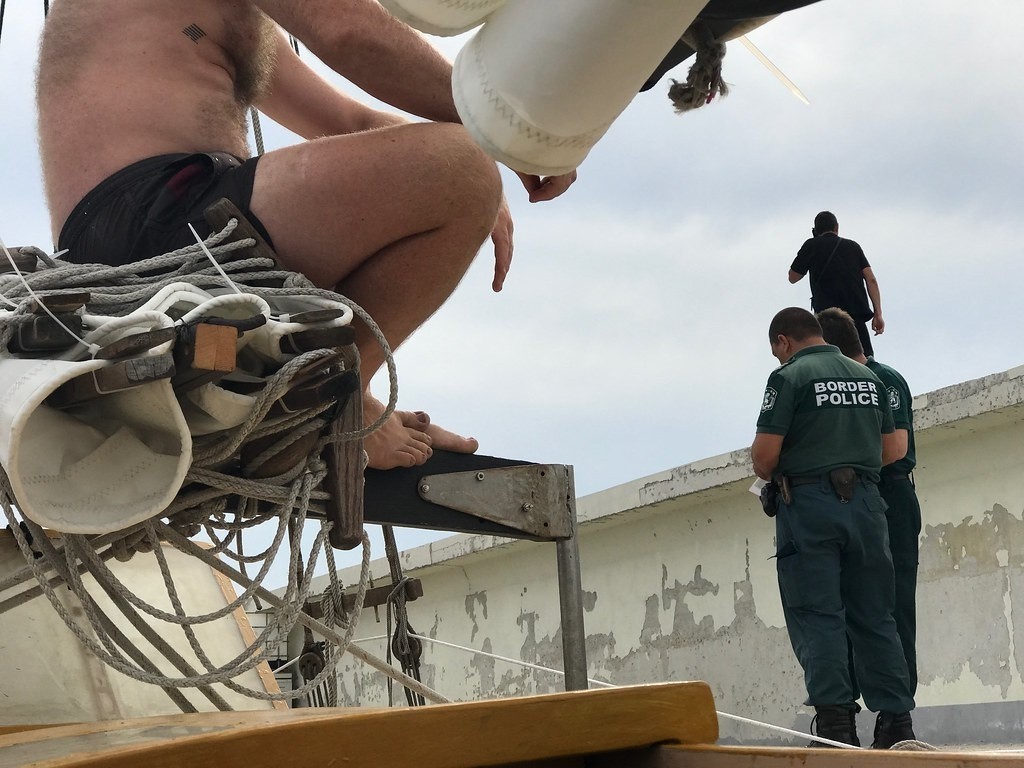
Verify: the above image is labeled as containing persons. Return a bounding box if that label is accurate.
[787,211,887,358]
[751,309,916,749]
[39,0,579,474]
[816,307,923,701]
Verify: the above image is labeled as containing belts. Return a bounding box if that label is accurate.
[788,471,863,488]
[879,469,910,482]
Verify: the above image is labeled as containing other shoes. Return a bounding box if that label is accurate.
[804,705,861,751]
[868,711,917,751]
